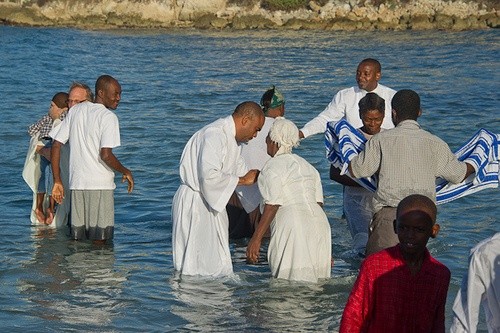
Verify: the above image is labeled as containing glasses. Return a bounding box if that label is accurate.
[65,99,87,104]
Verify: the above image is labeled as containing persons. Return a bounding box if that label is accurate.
[171,101,265,278]
[338,194,451,333]
[247,115,334,282]
[28,92,70,225]
[330,92,390,261]
[449,233,500,333]
[51,75,134,242]
[221,87,285,239]
[297,58,398,141]
[29,81,94,228]
[348,89,488,257]
